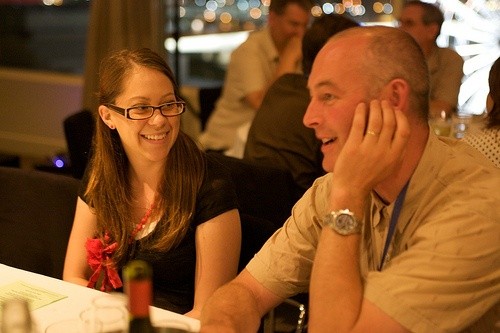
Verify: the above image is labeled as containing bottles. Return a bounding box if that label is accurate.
[124,261,154,333]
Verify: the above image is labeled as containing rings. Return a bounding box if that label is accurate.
[367,131,379,137]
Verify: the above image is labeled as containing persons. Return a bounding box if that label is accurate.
[241,14,363,197]
[198,26,500,333]
[397,1,465,120]
[203,1,311,159]
[458,57,500,166]
[61,45,244,318]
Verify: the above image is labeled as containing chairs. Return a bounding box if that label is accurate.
[0,166,80,280]
[63,109,96,179]
[199,88,223,133]
[204,150,305,333]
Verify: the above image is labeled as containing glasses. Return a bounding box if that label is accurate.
[106,96,186,119]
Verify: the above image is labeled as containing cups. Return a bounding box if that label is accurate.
[1,298,32,332]
[44,293,191,333]
[430,110,473,140]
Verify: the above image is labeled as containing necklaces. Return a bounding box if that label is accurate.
[133,210,149,232]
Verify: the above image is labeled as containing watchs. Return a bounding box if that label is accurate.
[320,209,364,235]
